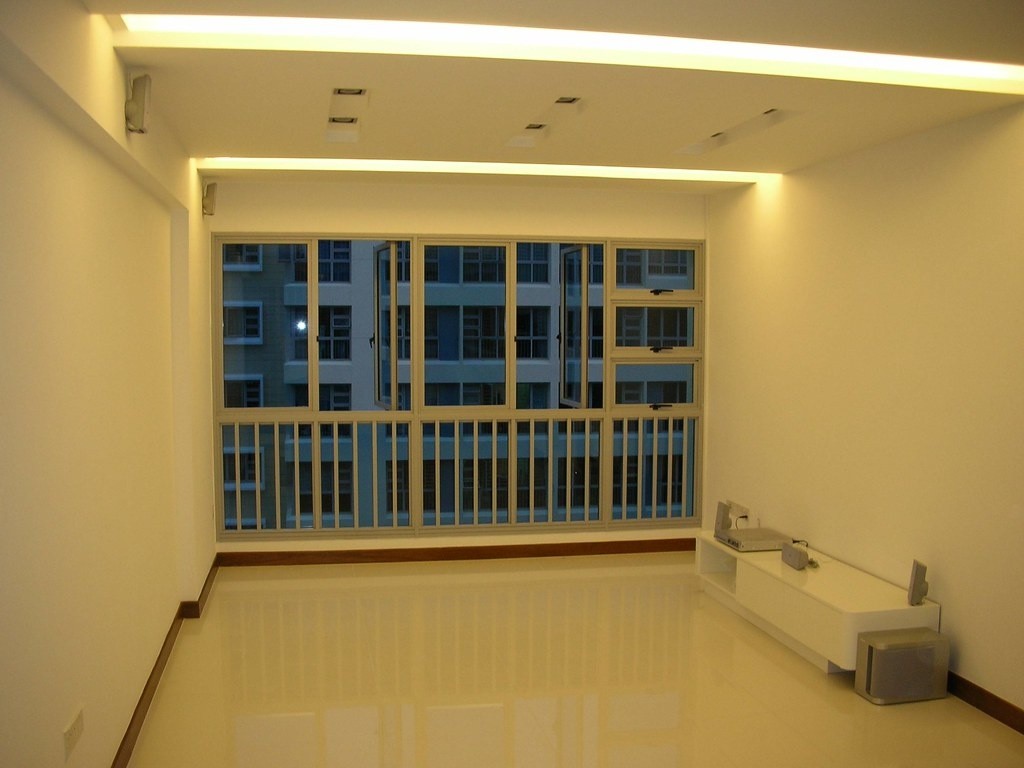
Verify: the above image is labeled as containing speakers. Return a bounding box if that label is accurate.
[126,74,153,135]
[908,560,928,604]
[781,541,809,570]
[853,626,951,706]
[203,183,217,215]
[714,502,732,539]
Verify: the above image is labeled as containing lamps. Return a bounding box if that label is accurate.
[201,182,216,216]
[124,74,151,134]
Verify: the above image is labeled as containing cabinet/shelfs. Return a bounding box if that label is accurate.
[695,530,941,674]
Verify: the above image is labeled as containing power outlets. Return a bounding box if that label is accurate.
[726,500,749,522]
